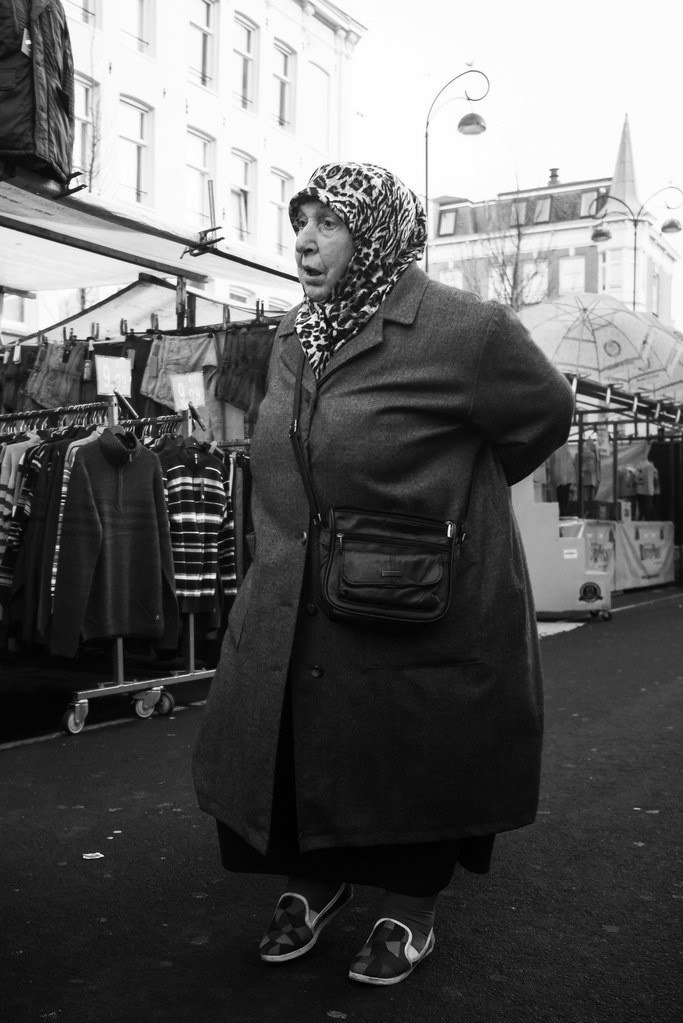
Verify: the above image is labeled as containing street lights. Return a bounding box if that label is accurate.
[425,70,489,276]
[588,185,682,311]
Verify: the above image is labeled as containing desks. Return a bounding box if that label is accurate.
[559,520,676,597]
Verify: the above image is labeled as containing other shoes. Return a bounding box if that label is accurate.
[347,917,438,984]
[257,883,354,962]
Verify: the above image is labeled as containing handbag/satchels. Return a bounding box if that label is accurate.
[318,506,458,624]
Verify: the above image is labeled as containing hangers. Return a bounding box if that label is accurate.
[89,329,124,351]
[231,314,281,335]
[156,312,214,341]
[0,402,183,450]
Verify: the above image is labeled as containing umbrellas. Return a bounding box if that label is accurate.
[510,292,683,410]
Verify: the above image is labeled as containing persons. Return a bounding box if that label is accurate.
[190,162,574,985]
